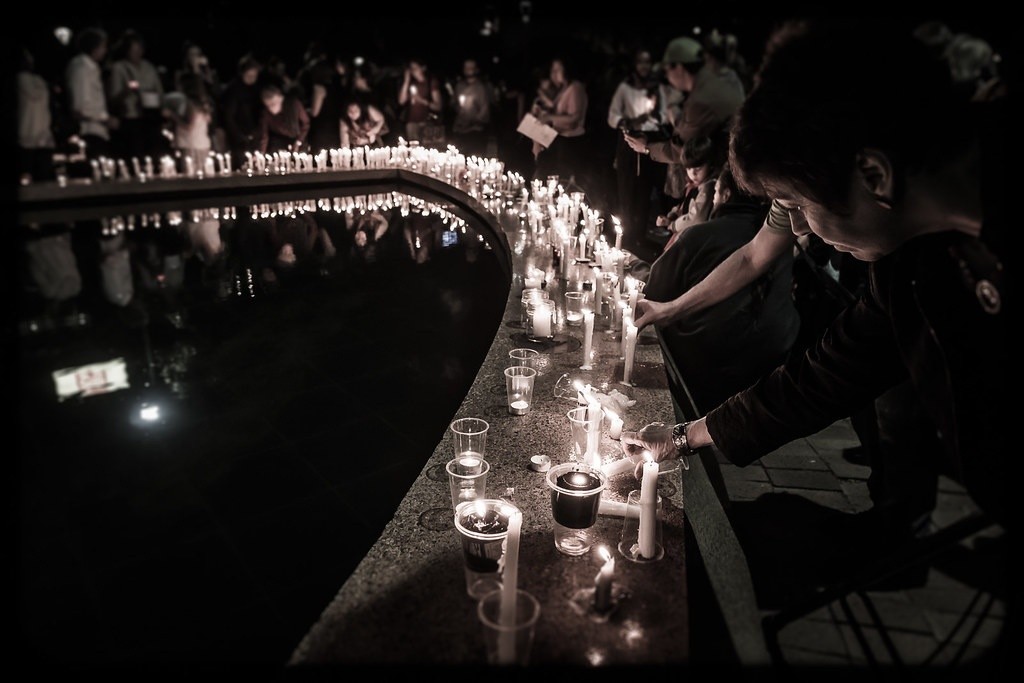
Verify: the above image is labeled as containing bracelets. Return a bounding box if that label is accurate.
[645,143,651,155]
[672,422,698,456]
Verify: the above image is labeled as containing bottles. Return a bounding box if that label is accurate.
[521,289,548,329]
[526,299,556,343]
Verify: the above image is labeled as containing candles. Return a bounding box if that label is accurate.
[102,192,492,252]
[54,133,460,189]
[460,150,661,660]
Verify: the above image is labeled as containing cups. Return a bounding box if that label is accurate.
[567,407,605,464]
[477,588,540,665]
[618,490,665,564]
[596,271,620,304]
[453,499,523,600]
[610,294,629,331]
[445,457,489,518]
[564,292,586,326]
[450,417,490,476]
[508,348,538,369]
[504,366,537,416]
[546,462,607,556]
[626,278,646,303]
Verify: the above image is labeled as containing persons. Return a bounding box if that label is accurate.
[18,13,1024,590]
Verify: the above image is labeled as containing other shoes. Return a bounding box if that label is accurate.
[842,507,930,589]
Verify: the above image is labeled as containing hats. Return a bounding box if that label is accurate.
[651,37,706,73]
